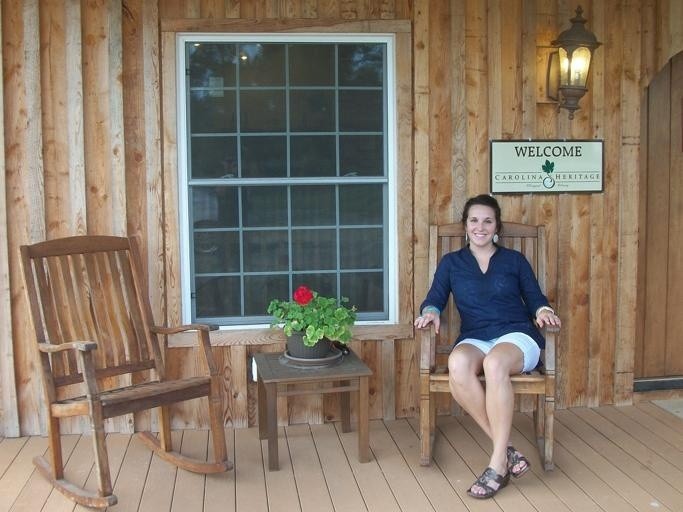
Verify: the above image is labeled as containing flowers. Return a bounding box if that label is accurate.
[267,285,358,348]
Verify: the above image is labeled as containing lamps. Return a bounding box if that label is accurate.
[535,4,602,118]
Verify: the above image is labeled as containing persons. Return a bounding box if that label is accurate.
[411,192,564,500]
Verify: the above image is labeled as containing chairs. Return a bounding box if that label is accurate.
[418,219,555,472]
[13,235,234,509]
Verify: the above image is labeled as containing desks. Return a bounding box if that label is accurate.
[255,342,371,472]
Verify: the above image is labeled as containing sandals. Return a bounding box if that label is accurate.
[466,468,510,499]
[507,446,531,478]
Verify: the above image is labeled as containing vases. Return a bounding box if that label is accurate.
[281,333,344,370]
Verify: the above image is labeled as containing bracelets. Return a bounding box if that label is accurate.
[422,307,440,313]
